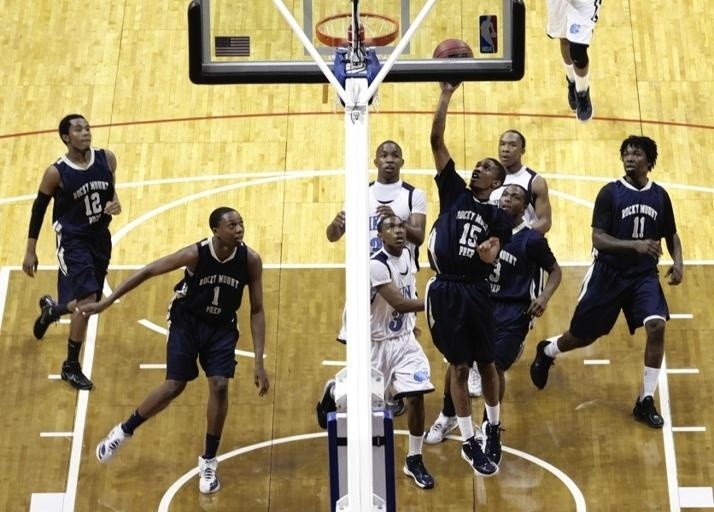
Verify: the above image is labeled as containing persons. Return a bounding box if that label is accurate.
[531,138,683,428]
[544,0,604,120]
[22,109,123,390]
[74,208,270,494]
[429,185,563,450]
[325,139,425,345]
[312,214,435,491]
[467,127,550,402]
[428,78,508,476]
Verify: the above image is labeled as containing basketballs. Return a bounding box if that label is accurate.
[432,40,473,58]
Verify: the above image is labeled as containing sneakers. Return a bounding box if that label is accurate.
[566,75,593,123]
[633,396,664,429]
[529,340,555,390]
[198,454,220,495]
[96,422,134,465]
[61,361,93,390]
[34,295,60,340]
[316,360,505,490]
